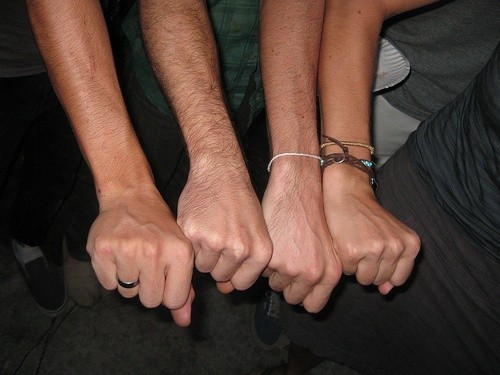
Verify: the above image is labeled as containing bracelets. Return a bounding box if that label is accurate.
[266,153,323,175]
[319,140,376,155]
[319,135,381,195]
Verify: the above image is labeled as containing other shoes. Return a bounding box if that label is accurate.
[244,363,289,375]
[62,238,100,308]
[11,243,66,313]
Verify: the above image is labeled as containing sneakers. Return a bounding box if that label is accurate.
[252,284,283,351]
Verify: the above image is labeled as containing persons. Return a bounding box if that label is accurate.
[0,0,500,351]
[260,0,500,375]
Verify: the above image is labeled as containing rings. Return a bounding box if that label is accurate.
[117,277,138,289]
